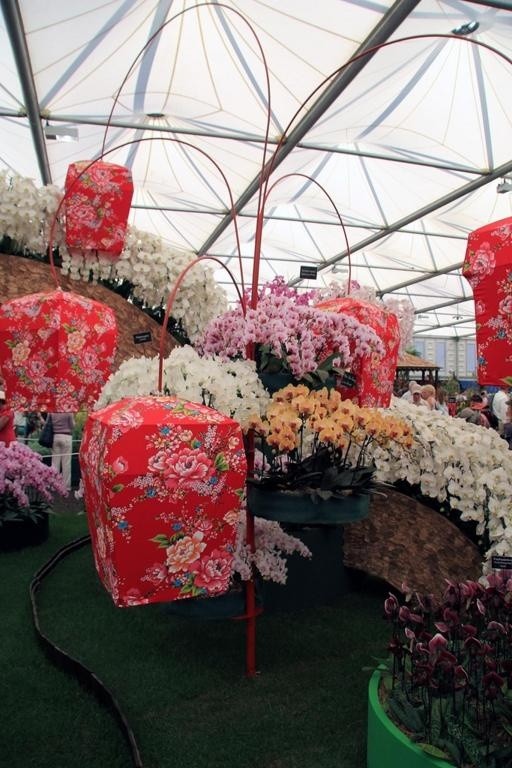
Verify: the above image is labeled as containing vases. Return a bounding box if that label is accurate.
[266,482,376,531]
[0,508,56,546]
[366,638,506,765]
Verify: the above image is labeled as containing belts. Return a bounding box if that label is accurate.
[53,431,72,437]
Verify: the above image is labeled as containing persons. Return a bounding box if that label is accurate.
[392,375,511,450]
[23,409,47,445]
[0,389,17,449]
[42,412,77,491]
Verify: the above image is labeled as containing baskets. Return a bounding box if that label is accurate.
[338,478,487,608]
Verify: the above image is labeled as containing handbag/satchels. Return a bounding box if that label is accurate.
[38,414,55,447]
[14,425,26,434]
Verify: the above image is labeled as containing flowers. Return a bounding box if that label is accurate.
[0,441,69,513]
[368,390,510,586]
[237,384,421,467]
[90,344,274,453]
[384,562,512,754]
[2,163,226,343]
[194,273,416,383]
